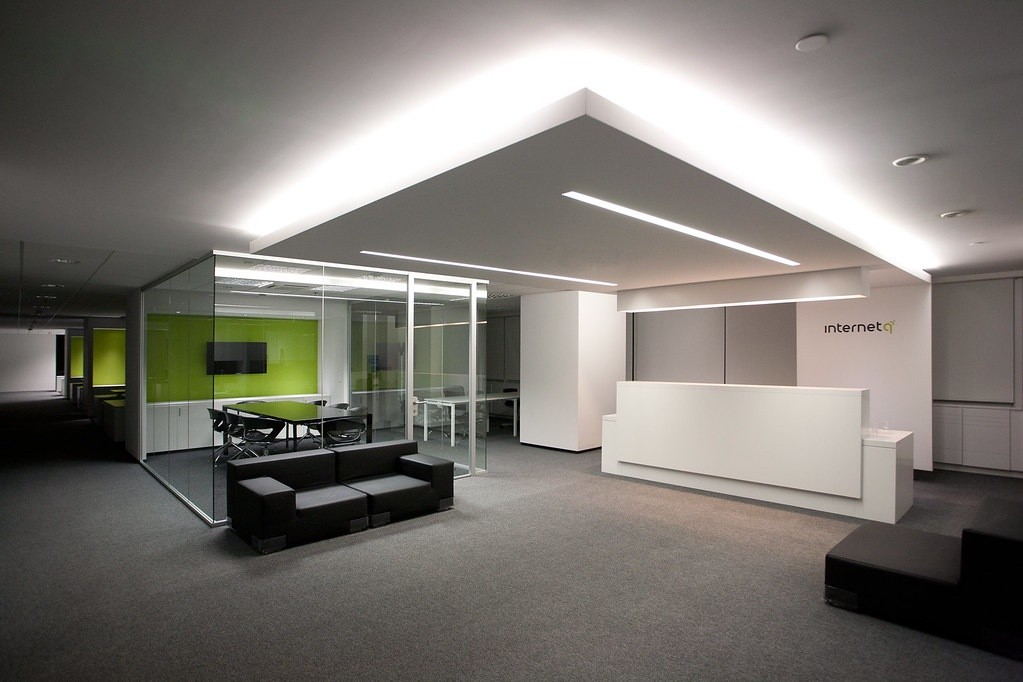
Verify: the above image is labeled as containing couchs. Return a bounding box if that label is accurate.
[227,439,367,554]
[328,439,455,528]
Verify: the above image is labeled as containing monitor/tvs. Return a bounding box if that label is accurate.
[206,342,267,376]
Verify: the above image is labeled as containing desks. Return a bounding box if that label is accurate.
[223,401,372,455]
[424,391,520,446]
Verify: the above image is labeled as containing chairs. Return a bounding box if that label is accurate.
[206,400,367,467]
[443,386,483,438]
[502,388,520,427]
[823,496,1023,663]
